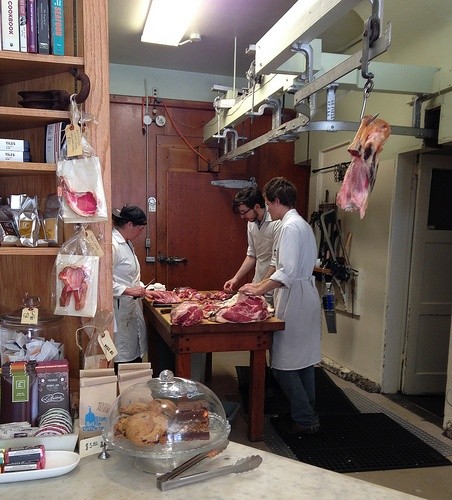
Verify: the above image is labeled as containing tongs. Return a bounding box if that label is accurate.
[156,438,263,491]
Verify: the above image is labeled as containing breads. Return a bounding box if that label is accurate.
[113,398,210,447]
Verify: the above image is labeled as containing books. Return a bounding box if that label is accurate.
[0,0,65,56]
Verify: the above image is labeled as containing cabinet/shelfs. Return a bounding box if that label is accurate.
[0,0,115,393]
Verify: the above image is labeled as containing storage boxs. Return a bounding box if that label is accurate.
[36,360,69,416]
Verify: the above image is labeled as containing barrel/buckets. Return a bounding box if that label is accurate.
[0,293,64,364]
[0,360,39,427]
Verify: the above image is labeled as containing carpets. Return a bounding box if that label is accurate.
[237,365,451,474]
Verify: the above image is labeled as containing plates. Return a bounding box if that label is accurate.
[0,451,81,484]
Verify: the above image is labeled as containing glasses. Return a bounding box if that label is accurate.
[238,204,256,216]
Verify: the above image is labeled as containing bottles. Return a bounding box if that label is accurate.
[81,309,114,370]
[323,282,336,316]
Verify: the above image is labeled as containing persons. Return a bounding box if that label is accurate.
[112,205,163,378]
[224,177,322,430]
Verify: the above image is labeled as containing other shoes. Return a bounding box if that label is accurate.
[291,422,319,436]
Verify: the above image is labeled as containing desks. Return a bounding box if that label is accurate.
[0,420,429,500]
[144,290,286,442]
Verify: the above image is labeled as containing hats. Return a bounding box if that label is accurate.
[111,206,148,225]
